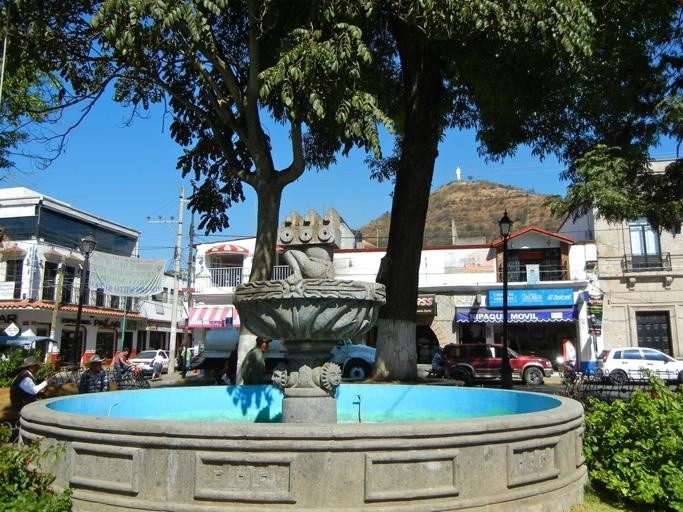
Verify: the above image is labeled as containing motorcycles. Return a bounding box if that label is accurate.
[425,362,472,386]
[556,355,577,385]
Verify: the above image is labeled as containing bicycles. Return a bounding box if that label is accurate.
[108,365,153,390]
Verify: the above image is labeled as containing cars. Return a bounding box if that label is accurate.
[125,349,178,374]
[596,346,683,385]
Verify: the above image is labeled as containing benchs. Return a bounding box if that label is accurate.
[0,378,122,450]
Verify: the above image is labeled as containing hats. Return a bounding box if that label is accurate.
[85,355,103,364]
[20,356,40,368]
[256,335,273,342]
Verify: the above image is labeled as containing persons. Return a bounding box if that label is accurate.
[112,347,134,385]
[241,337,273,382]
[79,354,110,393]
[561,335,576,385]
[430,347,445,376]
[280,245,336,281]
[10,356,59,413]
[151,350,166,382]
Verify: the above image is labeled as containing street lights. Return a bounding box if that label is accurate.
[73,232,97,367]
[497,208,513,389]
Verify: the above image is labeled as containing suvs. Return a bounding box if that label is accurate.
[444,342,554,385]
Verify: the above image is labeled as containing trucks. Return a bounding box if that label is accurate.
[189,326,376,385]
